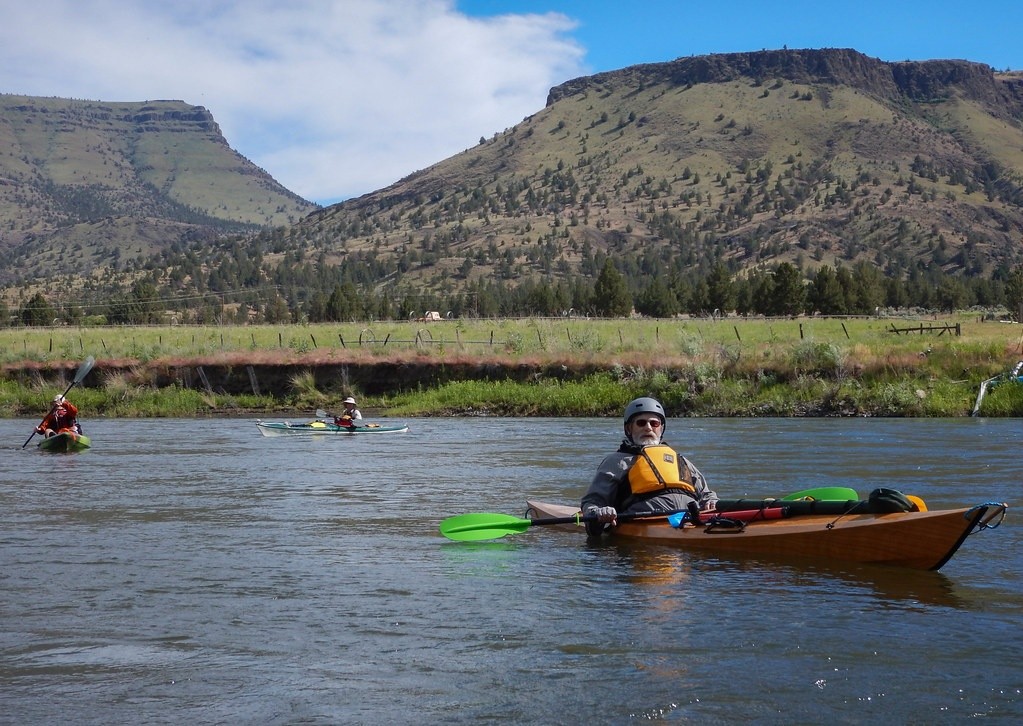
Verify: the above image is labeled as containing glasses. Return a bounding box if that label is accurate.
[631,419,662,427]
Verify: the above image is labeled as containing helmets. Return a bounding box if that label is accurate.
[623,396,666,442]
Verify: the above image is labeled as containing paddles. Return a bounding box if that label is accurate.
[315,408,367,428]
[437,485,862,543]
[21,354,95,449]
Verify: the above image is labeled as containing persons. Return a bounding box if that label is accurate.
[581,397,774,537]
[334,397,362,427]
[34,394,79,438]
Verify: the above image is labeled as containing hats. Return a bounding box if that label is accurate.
[50,395,66,406]
[342,397,358,410]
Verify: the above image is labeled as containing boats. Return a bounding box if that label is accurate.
[255,422,409,436]
[35,432,91,454]
[524,494,988,572]
